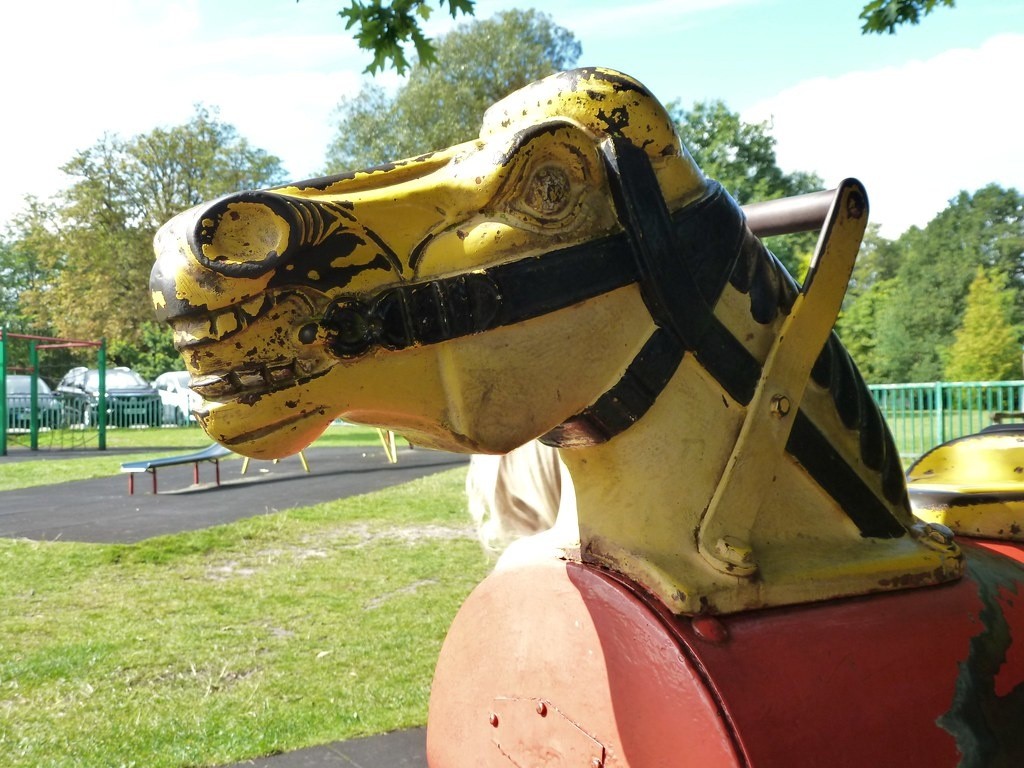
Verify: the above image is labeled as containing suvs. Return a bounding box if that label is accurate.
[54,367,163,427]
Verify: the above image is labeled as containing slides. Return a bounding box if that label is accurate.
[119,442,234,475]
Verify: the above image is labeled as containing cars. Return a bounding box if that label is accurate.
[5,373,62,429]
[153,371,226,428]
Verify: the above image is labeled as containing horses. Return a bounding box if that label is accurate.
[146,65,915,561]
